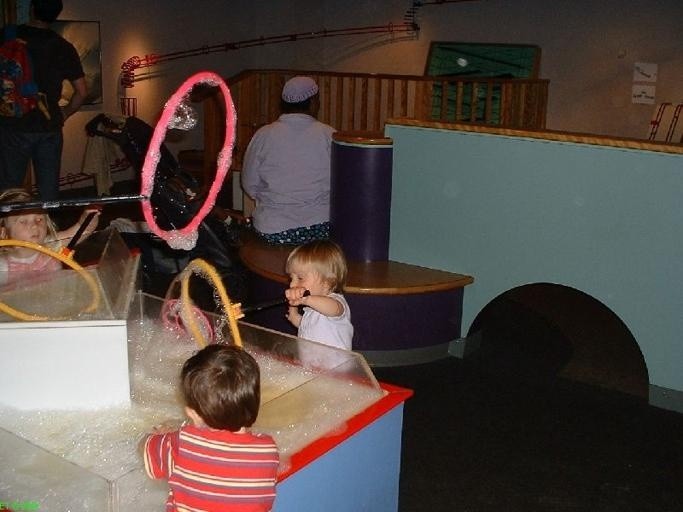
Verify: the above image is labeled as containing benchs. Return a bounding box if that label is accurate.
[238,238,474,368]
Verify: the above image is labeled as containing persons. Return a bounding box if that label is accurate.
[239,73,339,248]
[0,187,100,292]
[137,340,282,512]
[0,0,87,201]
[283,237,355,374]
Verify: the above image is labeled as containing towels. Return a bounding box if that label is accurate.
[82,136,114,197]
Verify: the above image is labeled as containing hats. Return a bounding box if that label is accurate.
[278,74,321,106]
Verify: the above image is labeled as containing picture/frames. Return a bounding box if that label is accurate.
[49,19,103,108]
